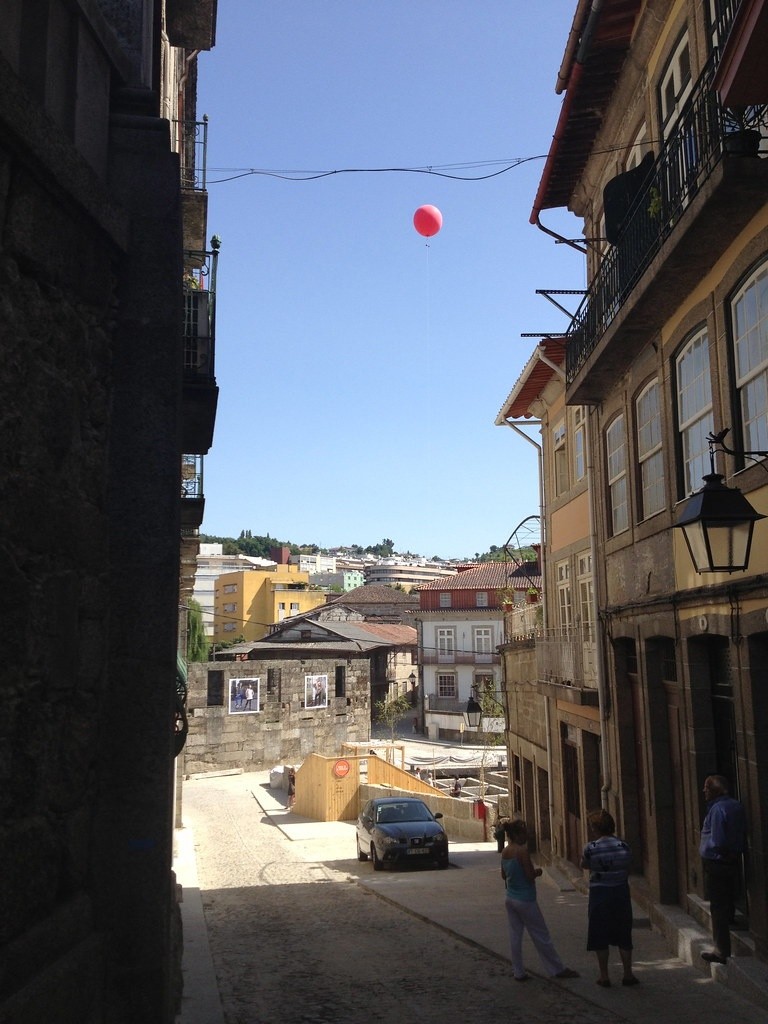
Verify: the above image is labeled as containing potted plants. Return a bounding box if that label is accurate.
[526,587,539,604]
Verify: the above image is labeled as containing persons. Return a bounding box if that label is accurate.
[426,773,434,788]
[285,767,296,808]
[311,679,324,707]
[452,774,462,798]
[699,775,749,965]
[234,681,255,711]
[499,819,579,982]
[369,749,377,756]
[579,808,639,989]
[414,767,421,779]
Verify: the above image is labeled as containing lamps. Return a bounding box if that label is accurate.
[671,442,768,578]
[462,685,484,727]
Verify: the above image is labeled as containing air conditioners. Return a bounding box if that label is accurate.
[182,289,209,373]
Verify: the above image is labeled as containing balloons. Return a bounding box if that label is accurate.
[413,204,443,238]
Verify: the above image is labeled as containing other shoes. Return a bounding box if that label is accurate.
[513,952,728,988]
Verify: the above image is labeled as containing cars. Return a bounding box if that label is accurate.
[355,796,453,873]
